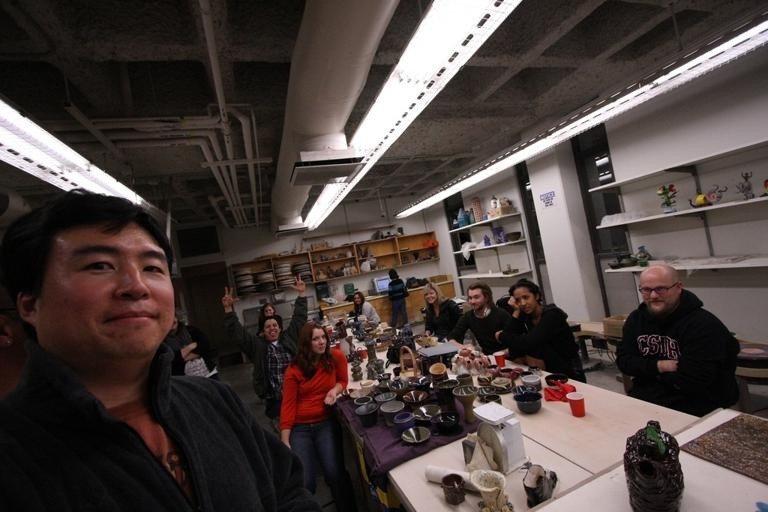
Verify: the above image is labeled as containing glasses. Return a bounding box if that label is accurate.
[638,281,678,294]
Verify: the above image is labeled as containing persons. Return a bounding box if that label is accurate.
[500,277,586,388]
[615,262,731,418]
[0,190,323,512]
[165,275,383,511]
[388,268,411,325]
[1,289,32,398]
[424,281,462,340]
[442,283,512,355]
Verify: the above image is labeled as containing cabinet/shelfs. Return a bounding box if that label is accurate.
[587,138,768,274]
[320,280,455,326]
[449,212,532,280]
[228,229,439,300]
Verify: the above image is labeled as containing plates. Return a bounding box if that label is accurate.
[233,262,313,296]
[544,373,567,386]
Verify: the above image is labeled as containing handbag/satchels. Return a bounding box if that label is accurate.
[406,277,419,288]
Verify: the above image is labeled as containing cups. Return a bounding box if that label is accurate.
[481,214,487,220]
[493,351,505,368]
[335,322,346,338]
[440,472,465,505]
[353,373,429,430]
[428,362,503,407]
[565,391,586,418]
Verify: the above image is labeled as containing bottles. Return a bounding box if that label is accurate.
[490,195,497,208]
[483,234,490,246]
[468,208,474,226]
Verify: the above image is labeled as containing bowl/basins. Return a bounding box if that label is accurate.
[416,337,438,348]
[400,405,459,445]
[503,231,521,241]
[490,367,542,414]
